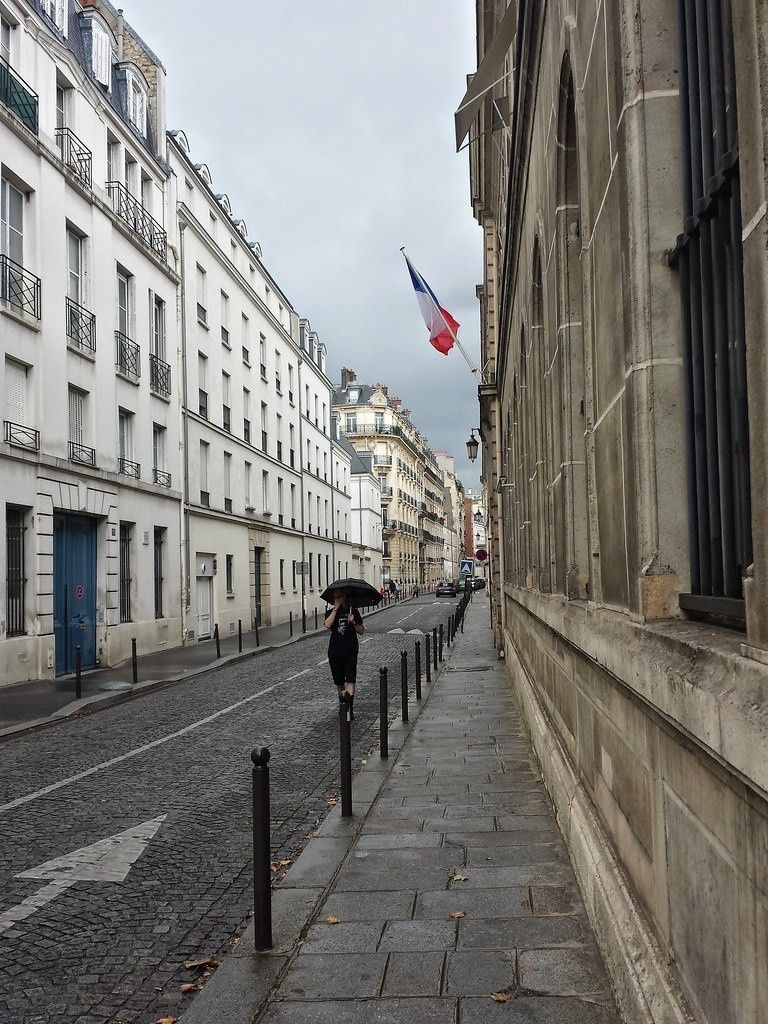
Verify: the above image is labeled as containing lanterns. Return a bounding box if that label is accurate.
[476,550,487,562]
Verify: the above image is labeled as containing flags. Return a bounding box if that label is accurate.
[402,246,460,355]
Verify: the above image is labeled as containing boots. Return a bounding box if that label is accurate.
[350,694,357,720]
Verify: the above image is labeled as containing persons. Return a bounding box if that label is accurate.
[412,584,419,598]
[323,589,364,721]
[389,580,395,599]
[395,579,400,598]
[380,588,384,596]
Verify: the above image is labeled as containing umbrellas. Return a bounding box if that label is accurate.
[320,578,384,608]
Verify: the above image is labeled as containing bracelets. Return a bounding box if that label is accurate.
[333,608,338,611]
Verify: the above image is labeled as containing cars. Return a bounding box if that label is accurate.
[452,576,486,593]
[436,582,457,597]
